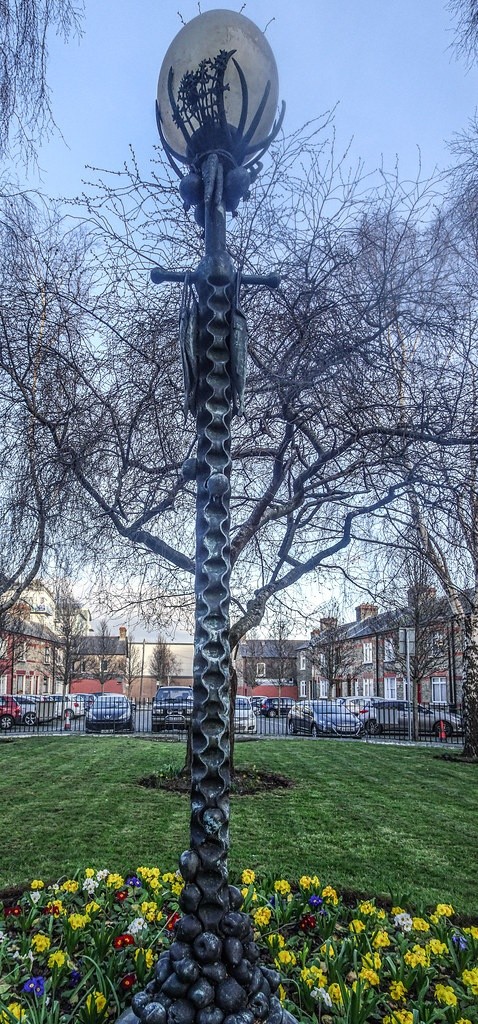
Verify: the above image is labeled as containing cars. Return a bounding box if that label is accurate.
[84,696,135,733]
[288,700,365,739]
[233,696,257,734]
[0,693,95,730]
[247,695,268,713]
[337,696,466,736]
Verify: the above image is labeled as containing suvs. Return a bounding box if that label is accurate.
[152,686,193,733]
[260,697,298,718]
